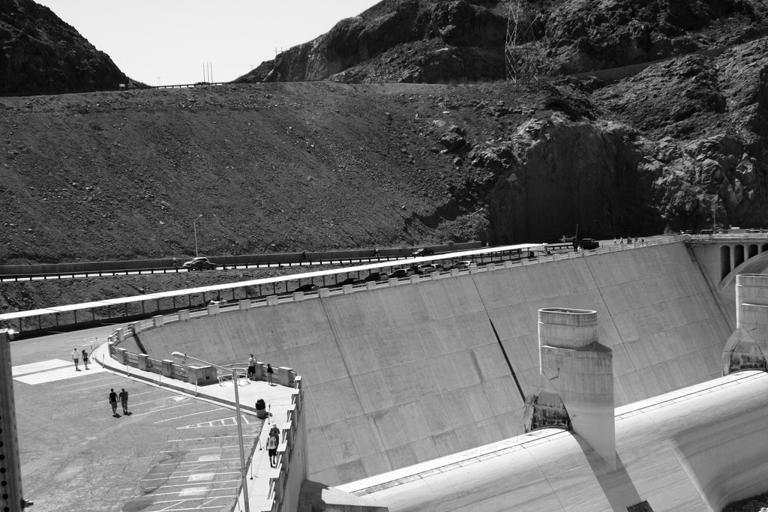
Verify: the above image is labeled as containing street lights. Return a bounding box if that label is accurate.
[193,215,203,256]
[172,351,249,512]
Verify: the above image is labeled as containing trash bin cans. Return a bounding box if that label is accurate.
[255,400,265,418]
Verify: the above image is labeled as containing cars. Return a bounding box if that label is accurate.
[183,257,216,269]
[412,248,434,256]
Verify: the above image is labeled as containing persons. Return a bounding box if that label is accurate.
[109,388,118,416]
[72,348,81,371]
[266,434,277,467]
[247,354,256,381]
[82,350,89,370]
[269,424,280,446]
[119,388,128,414]
[612,236,644,244]
[266,363,273,386]
